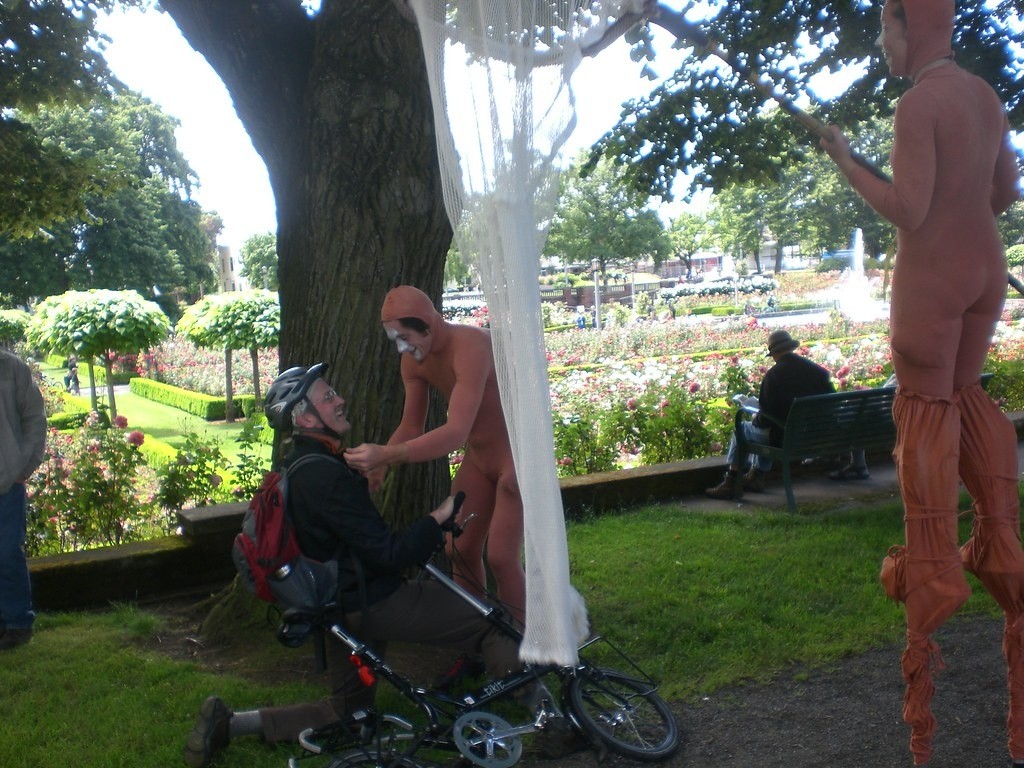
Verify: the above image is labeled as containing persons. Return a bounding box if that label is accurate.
[706,330,839,499]
[575,299,682,330]
[0,348,47,642]
[745,293,782,314]
[819,0,1024,628]
[184,363,495,768]
[829,370,900,478]
[65,355,80,394]
[26,357,42,381]
[344,285,530,669]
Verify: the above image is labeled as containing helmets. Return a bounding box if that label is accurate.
[264,362,329,433]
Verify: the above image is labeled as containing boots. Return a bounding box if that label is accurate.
[742,465,773,494]
[705,471,743,500]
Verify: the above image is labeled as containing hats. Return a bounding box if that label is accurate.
[765,330,800,356]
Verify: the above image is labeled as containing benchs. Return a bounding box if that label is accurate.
[733,373,995,511]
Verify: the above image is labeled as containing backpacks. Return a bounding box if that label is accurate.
[231,454,353,611]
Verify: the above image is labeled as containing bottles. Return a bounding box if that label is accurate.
[275,563,292,581]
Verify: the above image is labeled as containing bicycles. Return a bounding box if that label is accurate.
[272,489,681,768]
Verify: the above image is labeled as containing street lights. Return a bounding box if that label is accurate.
[591,257,603,330]
[630,263,638,299]
[261,265,269,288]
[732,256,739,309]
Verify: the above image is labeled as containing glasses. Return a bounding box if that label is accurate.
[306,388,339,410]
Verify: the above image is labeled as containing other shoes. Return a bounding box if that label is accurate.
[456,680,513,701]
[182,696,233,768]
[431,650,486,693]
[0,629,32,649]
[536,719,615,762]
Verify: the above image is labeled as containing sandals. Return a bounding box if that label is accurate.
[828,463,870,480]
